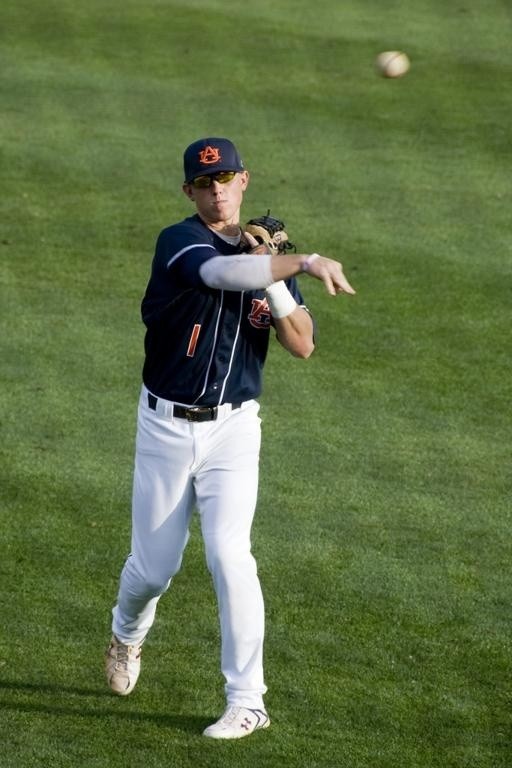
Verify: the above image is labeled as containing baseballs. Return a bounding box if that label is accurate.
[379,51,409,78]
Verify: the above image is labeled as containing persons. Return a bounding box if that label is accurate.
[101,137,356,741]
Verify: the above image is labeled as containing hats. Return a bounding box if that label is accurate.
[184,138,245,183]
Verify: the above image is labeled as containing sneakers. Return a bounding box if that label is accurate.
[203,705,270,739]
[104,633,147,696]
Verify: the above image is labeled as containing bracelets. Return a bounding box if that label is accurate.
[265,277,299,319]
[304,253,319,271]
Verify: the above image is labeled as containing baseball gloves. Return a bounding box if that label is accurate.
[240,217,293,254]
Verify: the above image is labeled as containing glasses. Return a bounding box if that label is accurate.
[193,171,234,188]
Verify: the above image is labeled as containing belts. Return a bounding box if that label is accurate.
[147,392,242,421]
